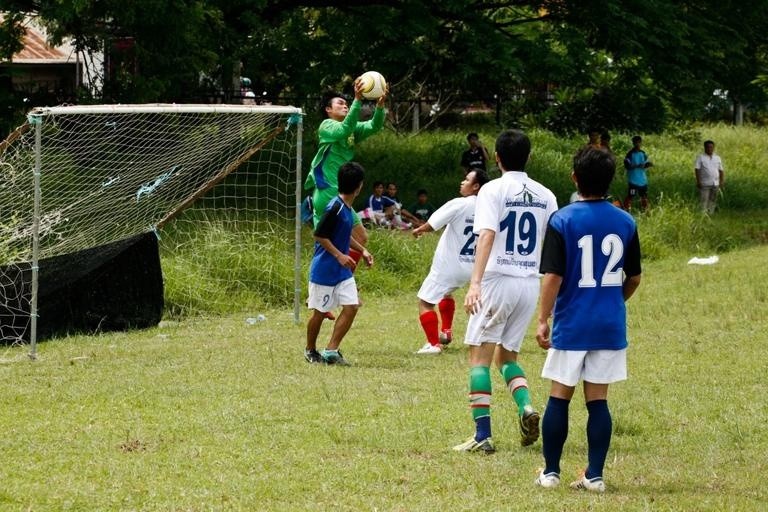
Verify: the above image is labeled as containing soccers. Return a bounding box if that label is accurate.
[359,71,386,100]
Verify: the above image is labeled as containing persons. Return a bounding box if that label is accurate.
[570,127,622,208]
[695,140,723,215]
[461,133,489,175]
[305,163,374,367]
[535,145,642,492]
[305,81,389,318]
[412,172,490,353]
[303,178,434,237]
[625,136,652,212]
[452,133,561,454]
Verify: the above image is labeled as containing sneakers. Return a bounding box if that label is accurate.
[412,341,444,355]
[303,345,353,368]
[305,296,336,321]
[439,330,454,344]
[569,475,606,493]
[517,404,542,446]
[534,468,561,489]
[451,432,496,455]
